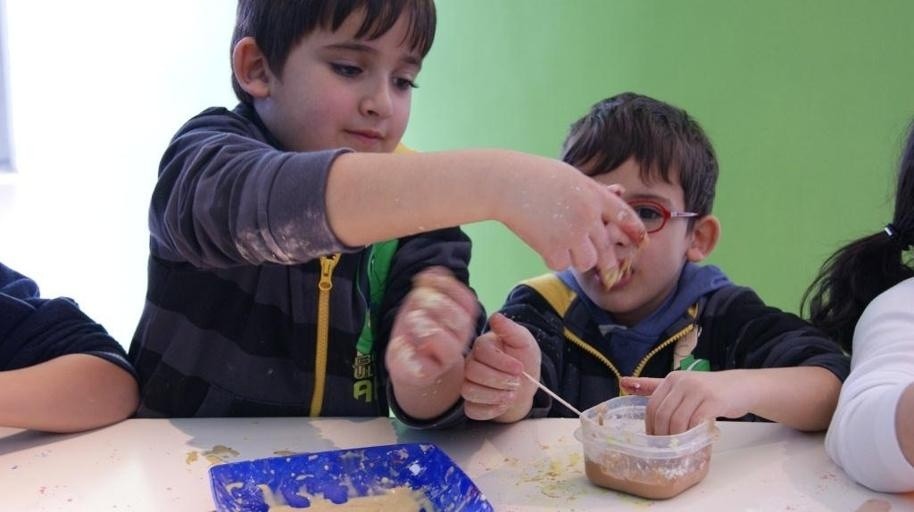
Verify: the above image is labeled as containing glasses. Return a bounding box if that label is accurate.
[621,192,711,235]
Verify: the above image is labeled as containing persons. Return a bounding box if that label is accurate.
[459,92,850,449]
[0,262,142,434]
[127,0,652,445]
[797,116,913,494]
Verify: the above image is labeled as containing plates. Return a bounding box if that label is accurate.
[208,440,493,512]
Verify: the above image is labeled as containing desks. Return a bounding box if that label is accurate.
[1,414,912,510]
